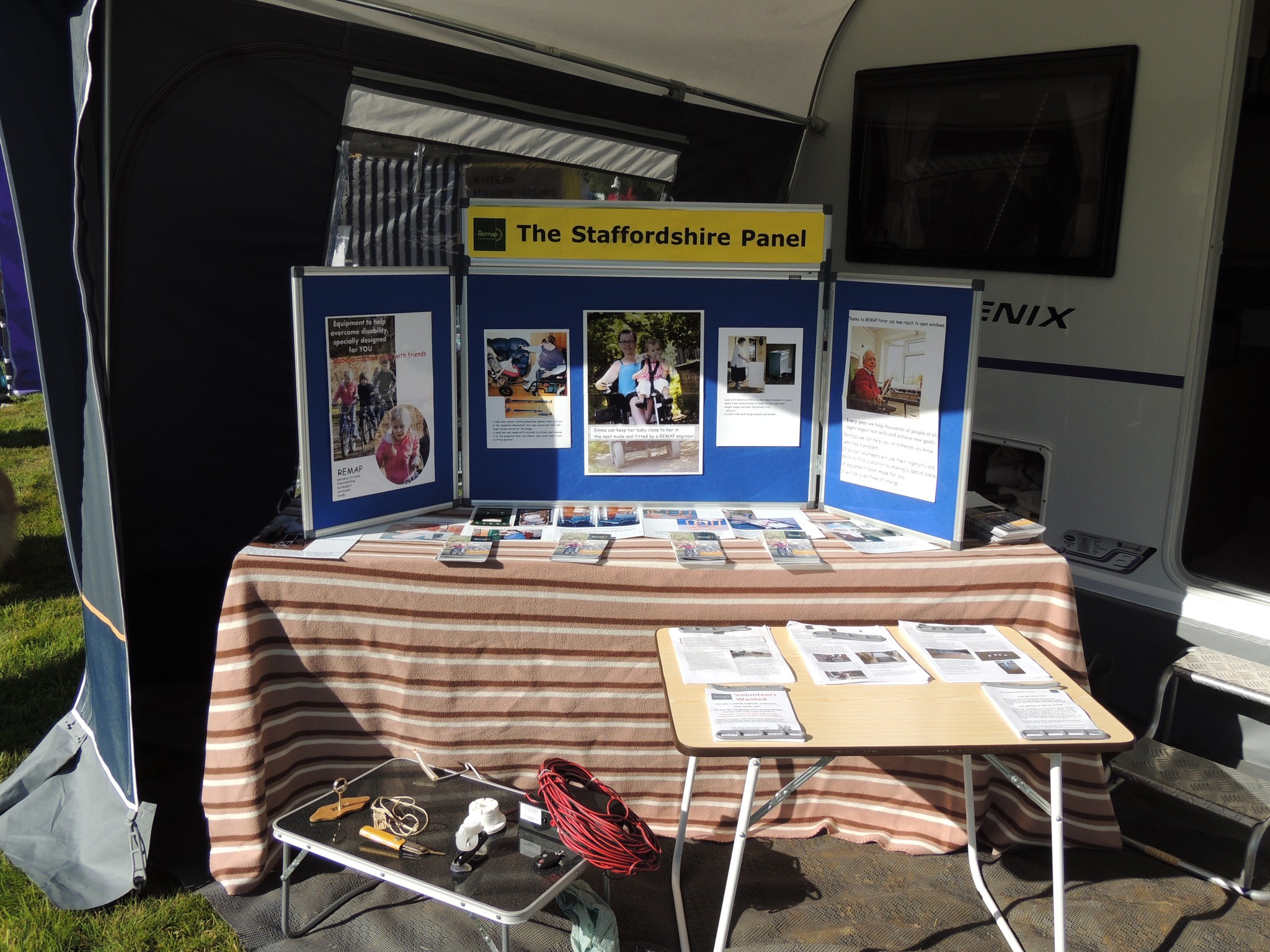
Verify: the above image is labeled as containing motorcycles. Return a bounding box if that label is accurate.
[594,358,675,425]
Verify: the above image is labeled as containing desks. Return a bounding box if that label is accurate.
[198,490,1125,906]
[273,756,613,952]
[655,620,1134,952]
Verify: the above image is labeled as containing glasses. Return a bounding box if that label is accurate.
[619,339,636,345]
[864,357,878,363]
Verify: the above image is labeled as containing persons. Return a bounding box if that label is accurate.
[730,337,751,390]
[678,543,700,556]
[504,529,526,539]
[851,350,893,404]
[448,543,466,554]
[595,329,673,424]
[770,541,794,554]
[563,542,581,553]
[985,514,1016,528]
[519,335,566,389]
[332,353,430,484]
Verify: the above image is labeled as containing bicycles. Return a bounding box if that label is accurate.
[333,402,357,457]
[357,394,377,444]
[377,390,394,423]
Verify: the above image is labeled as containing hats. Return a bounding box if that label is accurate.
[382,360,390,365]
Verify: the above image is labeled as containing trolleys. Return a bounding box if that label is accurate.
[769,350,790,381]
[746,361,765,391]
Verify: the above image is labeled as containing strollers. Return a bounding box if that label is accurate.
[486,345,522,396]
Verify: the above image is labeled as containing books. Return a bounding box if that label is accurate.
[964,505,1046,545]
[755,530,825,566]
[434,535,493,562]
[667,531,726,564]
[549,532,611,563]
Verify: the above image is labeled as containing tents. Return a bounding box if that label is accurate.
[0,0,1270,910]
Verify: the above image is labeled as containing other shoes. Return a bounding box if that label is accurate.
[373,407,379,413]
[355,436,361,441]
[373,420,378,430]
[523,384,537,389]
[663,395,673,405]
[523,377,537,381]
[358,427,362,431]
[636,399,648,409]
[338,436,341,443]
[735,385,744,390]
